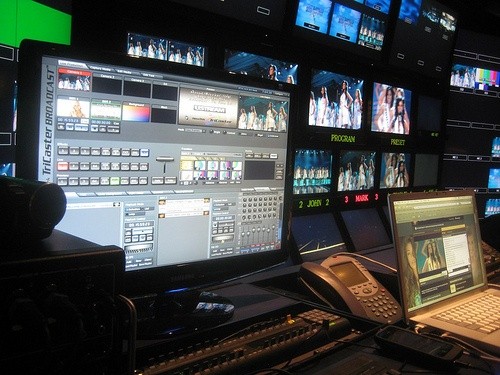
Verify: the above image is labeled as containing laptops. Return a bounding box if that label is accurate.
[387,190,500,357]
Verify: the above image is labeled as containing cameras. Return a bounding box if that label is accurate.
[0,175,68,242]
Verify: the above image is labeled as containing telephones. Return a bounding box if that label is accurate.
[296,252,405,324]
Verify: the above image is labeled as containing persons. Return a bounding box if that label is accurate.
[287,75,294,84]
[59,76,90,91]
[338,155,375,191]
[308,80,362,129]
[239,102,287,133]
[450,69,475,88]
[129,39,203,66]
[402,236,422,308]
[269,64,278,81]
[385,153,409,187]
[374,83,410,134]
[421,239,446,273]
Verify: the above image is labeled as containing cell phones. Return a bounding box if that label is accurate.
[375,325,463,364]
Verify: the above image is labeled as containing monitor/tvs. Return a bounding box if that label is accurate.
[15,0,500,340]
